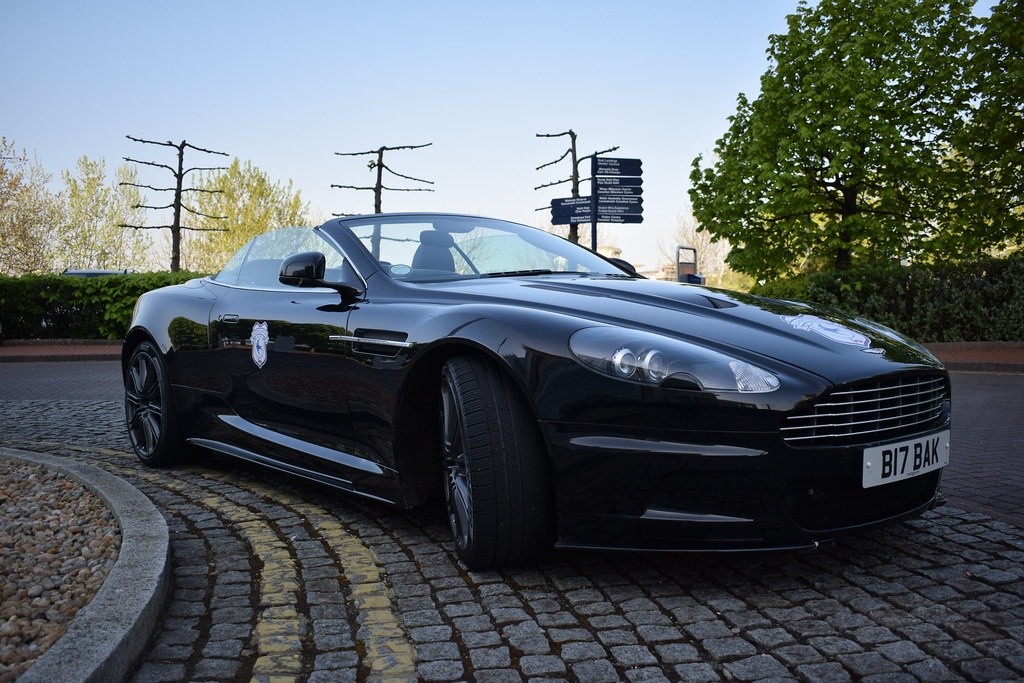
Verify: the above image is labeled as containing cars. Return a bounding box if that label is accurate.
[122,212,952,575]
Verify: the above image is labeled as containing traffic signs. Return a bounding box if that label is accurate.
[549,157,644,226]
